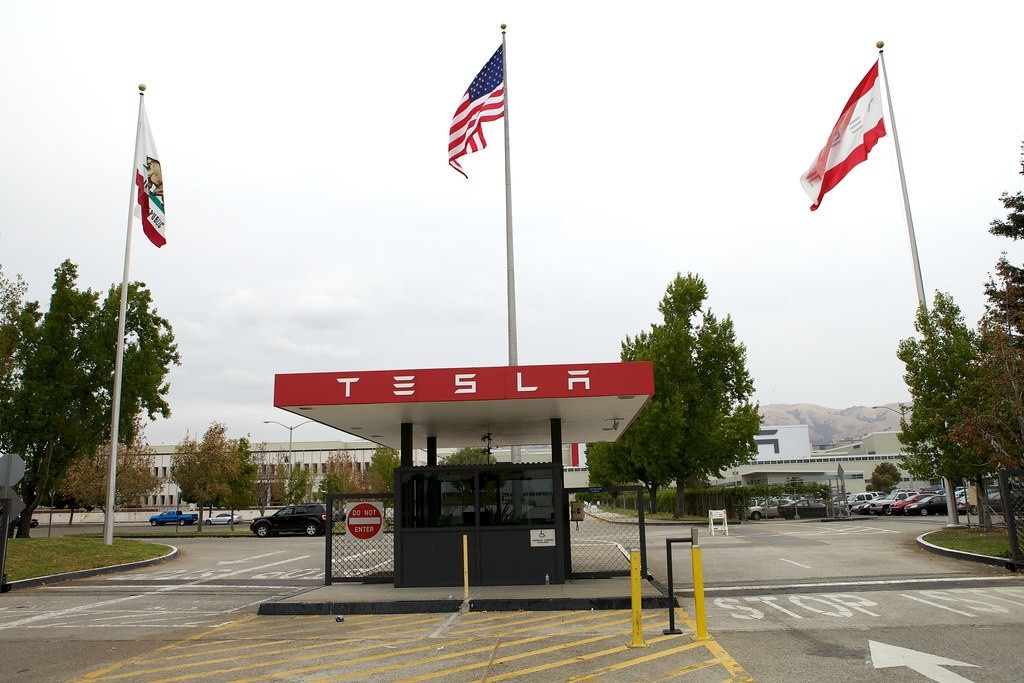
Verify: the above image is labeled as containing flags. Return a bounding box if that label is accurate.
[798,59,892,214]
[447,41,509,181]
[131,100,168,249]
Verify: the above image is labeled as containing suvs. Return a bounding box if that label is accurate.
[250,505,333,538]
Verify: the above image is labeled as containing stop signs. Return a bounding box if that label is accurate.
[347,503,383,540]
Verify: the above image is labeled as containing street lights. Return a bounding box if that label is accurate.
[263,420,314,503]
[872,405,916,492]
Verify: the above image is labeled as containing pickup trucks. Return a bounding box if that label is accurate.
[149,510,198,526]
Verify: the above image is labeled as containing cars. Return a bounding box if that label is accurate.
[833,487,1014,516]
[14,516,38,528]
[777,499,832,520]
[749,499,792,521]
[204,513,242,525]
